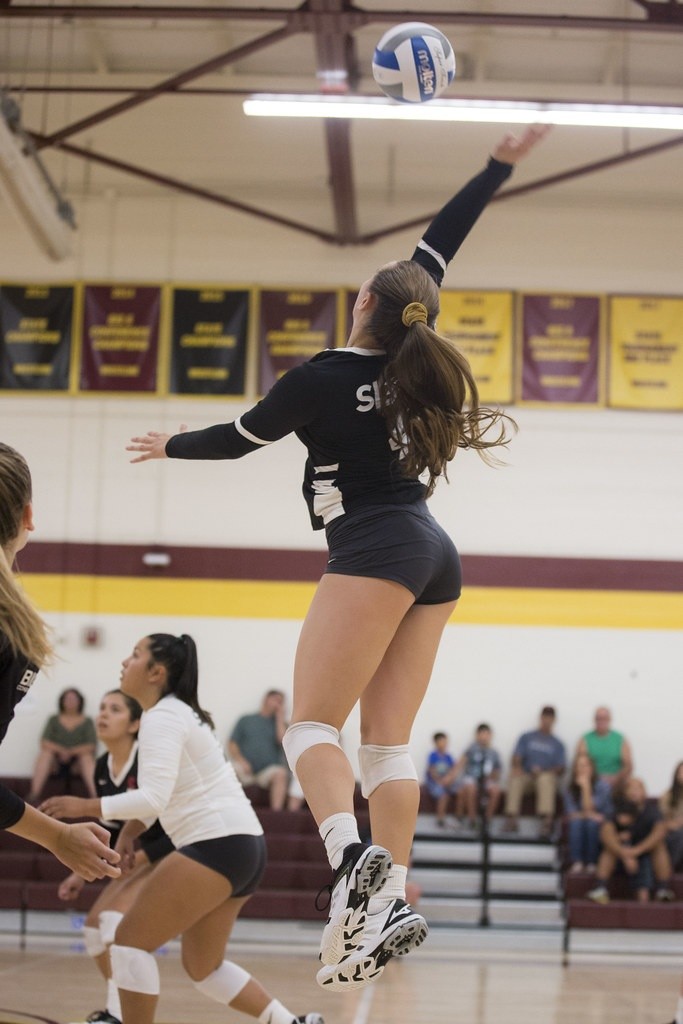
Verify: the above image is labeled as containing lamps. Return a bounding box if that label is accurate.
[241,91,682,134]
[0,112,80,257]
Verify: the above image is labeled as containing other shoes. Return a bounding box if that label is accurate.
[658,888,675,903]
[82,1009,121,1024]
[588,888,611,905]
[291,1011,324,1024]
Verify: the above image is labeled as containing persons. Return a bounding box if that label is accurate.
[425,725,501,829]
[57,689,178,1024]
[34,634,324,1024]
[127,122,556,989]
[0,442,123,882]
[27,688,98,803]
[559,708,683,903]
[502,707,567,840]
[228,691,304,812]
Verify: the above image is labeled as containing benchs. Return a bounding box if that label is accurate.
[1,773,682,929]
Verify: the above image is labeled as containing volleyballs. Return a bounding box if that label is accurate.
[372,21,457,104]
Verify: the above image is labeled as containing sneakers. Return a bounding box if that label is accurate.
[314,841,393,966]
[316,896,430,992]
